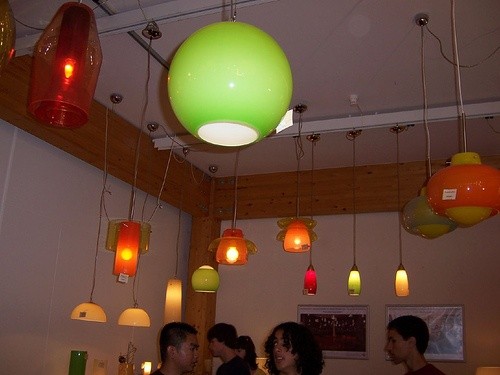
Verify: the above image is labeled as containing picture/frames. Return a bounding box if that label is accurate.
[386,303,465,362]
[297,304,371,360]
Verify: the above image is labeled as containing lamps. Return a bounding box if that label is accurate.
[191,165,220,294]
[303,133,322,296]
[169,1,294,148]
[113,20,163,276]
[426,1,500,229]
[118,123,160,328]
[346,128,363,296]
[388,126,410,296]
[165,148,190,322]
[400,13,457,240]
[28,0,103,129]
[208,147,259,266]
[71,94,124,322]
[275,105,319,253]
[143,361,151,375]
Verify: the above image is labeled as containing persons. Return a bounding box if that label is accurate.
[264,321,323,375]
[387,315,447,375]
[207,323,267,375]
[150,322,199,375]
